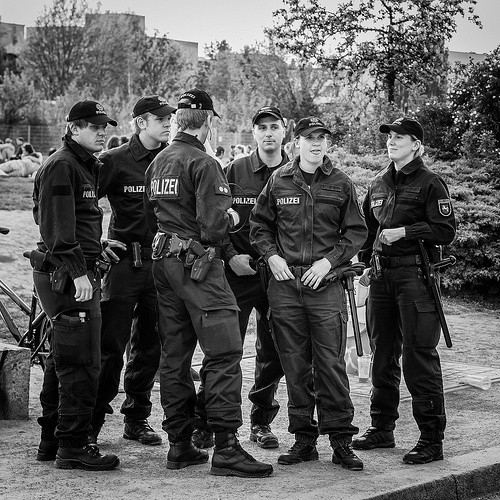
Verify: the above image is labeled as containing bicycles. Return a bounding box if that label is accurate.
[0,224,174,393]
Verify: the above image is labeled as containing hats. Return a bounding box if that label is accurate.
[131,95,177,117]
[293,116,333,137]
[252,106,284,126]
[177,88,221,118]
[380,116,425,143]
[66,100,118,126]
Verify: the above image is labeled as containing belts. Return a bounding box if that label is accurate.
[378,254,422,269]
[111,244,155,260]
[288,266,311,278]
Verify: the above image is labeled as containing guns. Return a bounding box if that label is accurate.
[256,256,268,292]
[45,250,69,294]
[189,241,216,282]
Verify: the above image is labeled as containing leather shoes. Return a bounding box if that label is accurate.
[208,446,273,479]
[251,424,280,448]
[164,442,208,470]
[36,440,59,461]
[332,445,363,470]
[191,429,214,447]
[122,418,162,445]
[351,426,395,449]
[403,436,444,464]
[278,440,320,465]
[52,446,119,471]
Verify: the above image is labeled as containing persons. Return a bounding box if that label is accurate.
[229,145,236,162]
[107,136,120,151]
[245,145,253,156]
[357,117,457,463]
[234,144,249,161]
[248,116,370,470]
[0,137,44,178]
[215,146,230,168]
[31,100,121,471]
[119,136,129,145]
[143,87,276,478]
[92,94,178,445]
[191,107,290,448]
